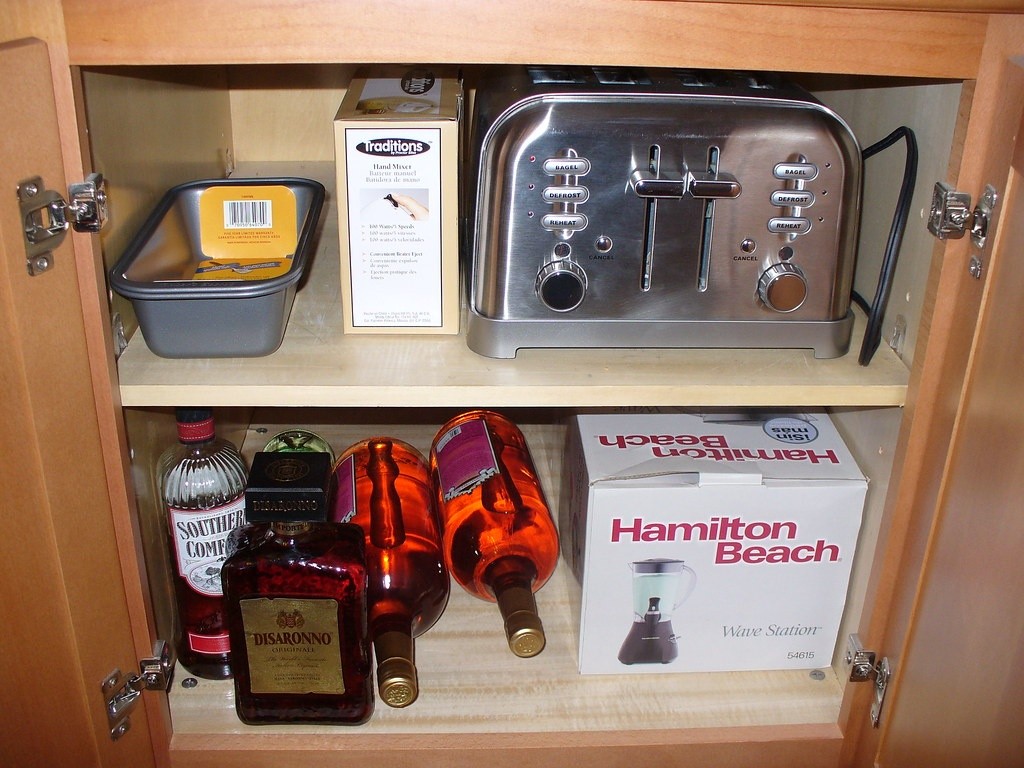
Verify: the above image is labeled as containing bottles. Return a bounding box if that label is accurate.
[430,410,561,658]
[220,451,374,726]
[330,436,452,708]
[156,409,249,680]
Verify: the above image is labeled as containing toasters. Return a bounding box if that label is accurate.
[467,65,918,366]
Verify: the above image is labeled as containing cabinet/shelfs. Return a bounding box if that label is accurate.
[0,0,1024,768]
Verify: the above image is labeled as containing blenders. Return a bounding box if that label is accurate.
[618,558,696,665]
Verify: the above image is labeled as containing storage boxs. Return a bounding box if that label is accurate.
[566,406,872,678]
[108,176,325,357]
[332,65,466,333]
